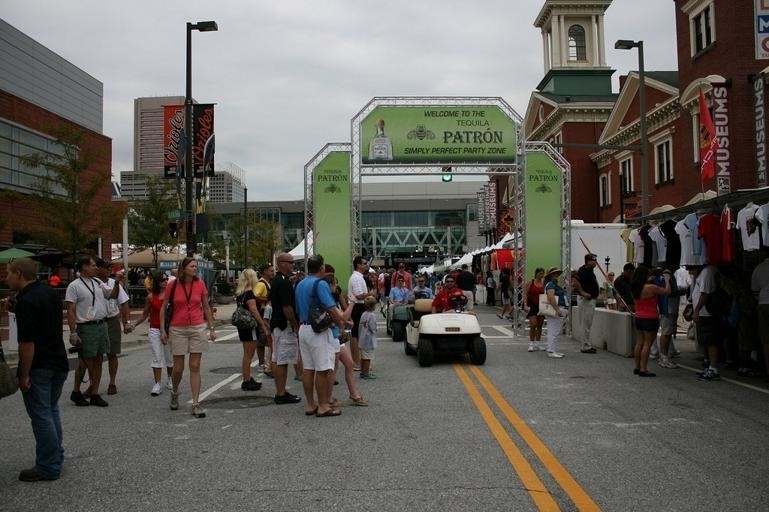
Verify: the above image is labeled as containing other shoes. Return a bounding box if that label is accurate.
[82,388,91,398]
[329,398,344,407]
[90,394,108,407]
[170,390,179,409]
[241,376,261,391]
[191,404,205,415]
[151,384,163,394]
[257,365,265,372]
[263,367,273,376]
[274,391,301,403]
[165,379,173,390]
[71,390,90,406]
[360,373,375,379]
[108,384,116,394]
[348,395,368,406]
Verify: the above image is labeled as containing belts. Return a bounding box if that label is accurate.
[76,319,103,326]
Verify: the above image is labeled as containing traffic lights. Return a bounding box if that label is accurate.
[441,165,452,182]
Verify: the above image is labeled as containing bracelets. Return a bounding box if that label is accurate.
[124,320,129,325]
[131,324,136,331]
[70,331,78,337]
[208,326,215,331]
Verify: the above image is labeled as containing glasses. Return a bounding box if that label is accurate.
[418,280,425,283]
[281,261,294,264]
[445,281,454,284]
[162,278,168,281]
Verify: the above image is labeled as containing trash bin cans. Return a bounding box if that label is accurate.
[8,311,19,351]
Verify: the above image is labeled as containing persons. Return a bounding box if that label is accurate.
[7,258,69,481]
[294,256,346,416]
[607,252,722,380]
[376,262,476,314]
[271,252,301,403]
[238,253,377,391]
[323,272,368,407]
[160,257,215,418]
[751,244,769,378]
[129,271,174,396]
[84,262,131,398]
[485,266,578,358]
[64,256,125,406]
[574,254,599,354]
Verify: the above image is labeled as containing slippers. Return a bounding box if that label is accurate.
[305,405,319,415]
[315,408,341,416]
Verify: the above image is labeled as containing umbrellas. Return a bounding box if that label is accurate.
[0,248,35,264]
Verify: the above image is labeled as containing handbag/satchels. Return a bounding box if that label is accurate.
[232,302,258,329]
[309,303,332,333]
[0,354,19,398]
[522,297,530,313]
[164,302,173,336]
[537,295,559,318]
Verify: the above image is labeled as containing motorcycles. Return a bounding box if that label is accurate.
[383,297,414,341]
[404,294,487,369]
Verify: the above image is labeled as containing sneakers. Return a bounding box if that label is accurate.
[633,351,720,381]
[19,466,58,481]
[528,341,596,358]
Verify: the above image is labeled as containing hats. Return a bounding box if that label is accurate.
[96,259,114,266]
[544,267,562,278]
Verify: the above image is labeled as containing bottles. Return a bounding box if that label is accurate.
[603,296,617,310]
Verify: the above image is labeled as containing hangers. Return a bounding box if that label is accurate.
[627,200,730,233]
[745,199,756,209]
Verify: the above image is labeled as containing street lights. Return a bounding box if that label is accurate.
[615,36,648,222]
[184,18,218,254]
